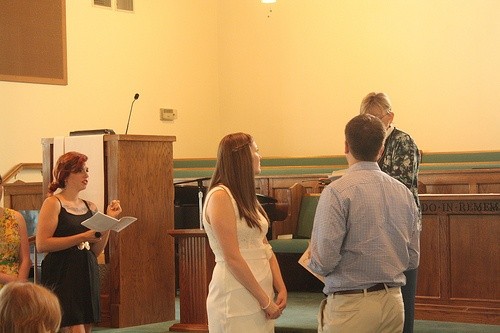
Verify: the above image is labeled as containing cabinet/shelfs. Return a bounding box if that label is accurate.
[413,192,500,326]
[41,134,176,328]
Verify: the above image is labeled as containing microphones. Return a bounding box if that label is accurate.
[126,93,139,134]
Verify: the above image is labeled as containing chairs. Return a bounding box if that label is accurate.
[269,196,321,253]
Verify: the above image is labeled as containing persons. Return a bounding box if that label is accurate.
[360,93,422,333]
[0,175,31,291]
[0,279,62,333]
[36,151,122,333]
[202,132,287,333]
[307,113,420,333]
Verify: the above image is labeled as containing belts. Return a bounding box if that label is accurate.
[326,281,403,293]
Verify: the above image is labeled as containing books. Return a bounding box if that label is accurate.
[277,233,311,239]
[298,242,325,284]
[81,210,138,233]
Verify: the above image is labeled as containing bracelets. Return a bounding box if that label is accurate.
[262,295,271,309]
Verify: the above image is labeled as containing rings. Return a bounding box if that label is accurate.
[95,232,101,238]
